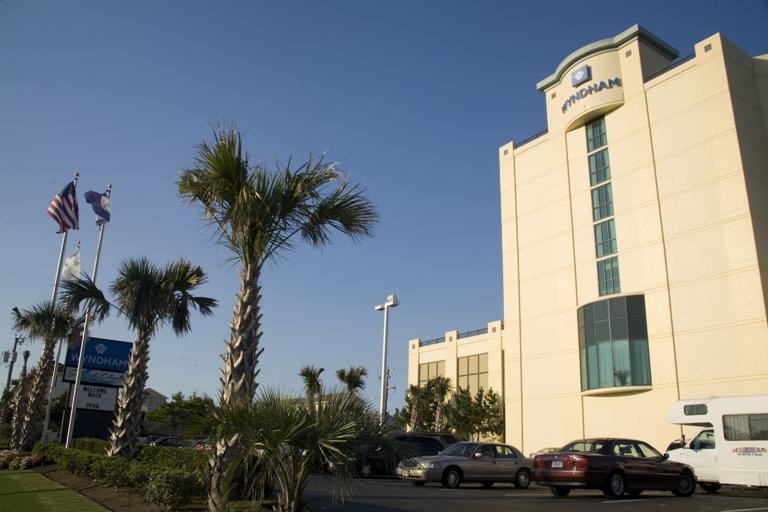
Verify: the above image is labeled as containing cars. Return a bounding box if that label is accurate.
[386,433,456,476]
[533,437,697,498]
[149,436,178,448]
[395,441,534,489]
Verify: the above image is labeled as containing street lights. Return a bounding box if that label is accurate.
[1,333,25,393]
[373,294,398,428]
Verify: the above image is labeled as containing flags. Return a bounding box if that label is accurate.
[47,180,79,233]
[85,190,111,225]
[59,248,81,281]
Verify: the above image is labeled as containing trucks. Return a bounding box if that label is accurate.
[663,394,768,494]
